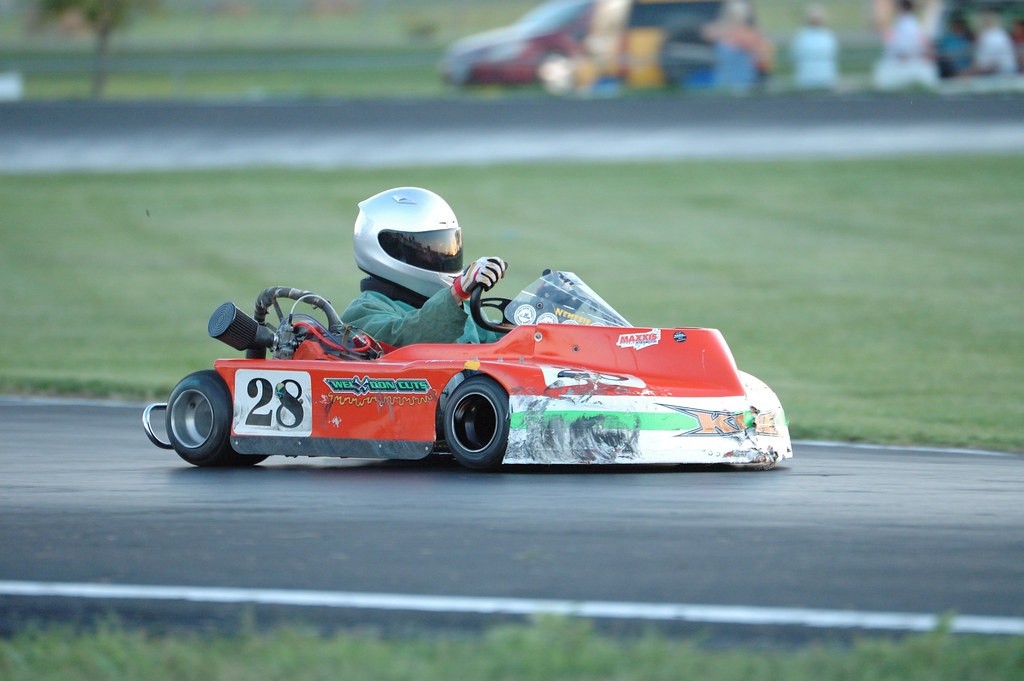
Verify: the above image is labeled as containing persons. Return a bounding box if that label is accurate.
[696,1,1020,94]
[338,185,587,349]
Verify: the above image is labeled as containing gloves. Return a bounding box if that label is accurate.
[453,257,509,301]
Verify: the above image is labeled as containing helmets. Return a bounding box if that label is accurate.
[352,187,464,298]
